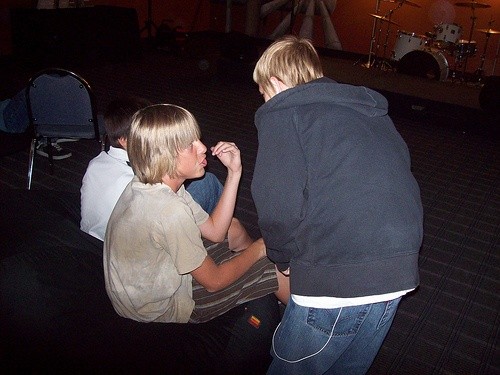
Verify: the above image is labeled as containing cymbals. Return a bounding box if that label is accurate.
[393,0,422,9]
[474,28,500,35]
[452,2,493,9]
[368,12,402,28]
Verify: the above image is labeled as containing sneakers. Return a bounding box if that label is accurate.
[51,138,79,143]
[36,141,72,160]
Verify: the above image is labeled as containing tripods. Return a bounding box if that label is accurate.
[353,0,405,71]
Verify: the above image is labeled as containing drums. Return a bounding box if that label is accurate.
[450,40,478,57]
[389,30,427,65]
[434,22,464,51]
[395,47,450,80]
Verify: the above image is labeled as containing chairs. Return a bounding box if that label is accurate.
[27,68,107,191]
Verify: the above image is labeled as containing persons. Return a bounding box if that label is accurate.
[103,105,290,323]
[251,34,424,375]
[80,98,223,242]
